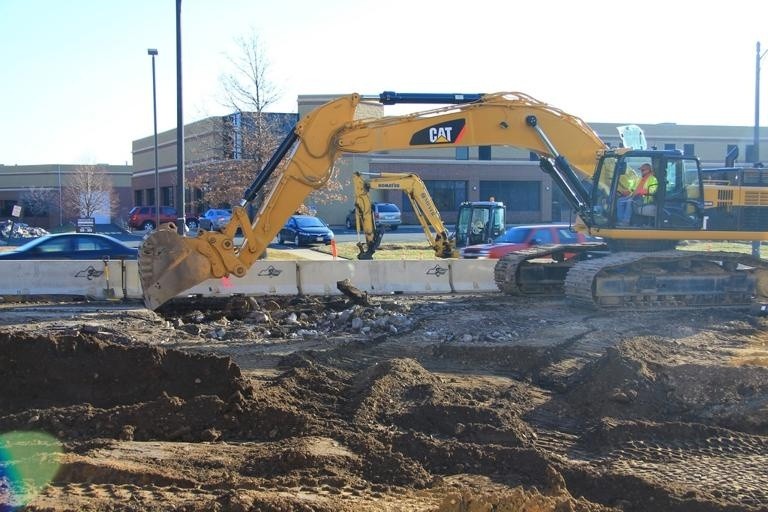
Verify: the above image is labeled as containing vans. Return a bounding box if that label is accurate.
[344,201,403,231]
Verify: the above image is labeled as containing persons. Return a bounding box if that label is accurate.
[616,164,658,227]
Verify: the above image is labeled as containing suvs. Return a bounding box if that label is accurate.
[126,204,202,230]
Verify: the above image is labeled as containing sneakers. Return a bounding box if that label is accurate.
[615,220,631,226]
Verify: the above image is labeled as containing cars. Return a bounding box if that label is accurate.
[275,215,337,249]
[0,229,140,262]
[458,218,603,261]
[196,206,242,233]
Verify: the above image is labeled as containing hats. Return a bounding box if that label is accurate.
[638,163,651,171]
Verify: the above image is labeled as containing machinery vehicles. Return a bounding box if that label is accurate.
[129,78,768,319]
[348,163,512,263]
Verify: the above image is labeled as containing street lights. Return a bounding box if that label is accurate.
[146,47,161,230]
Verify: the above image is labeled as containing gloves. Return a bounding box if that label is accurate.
[634,188,649,196]
[622,190,632,195]
